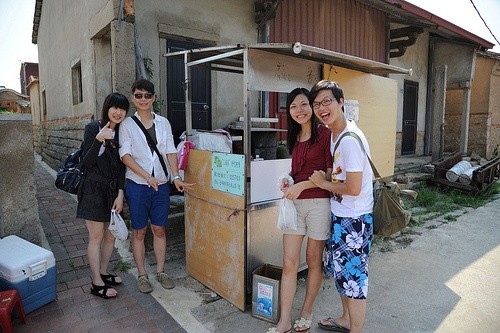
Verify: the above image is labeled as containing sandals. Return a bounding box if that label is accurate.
[90,281,118,299]
[101,272,123,285]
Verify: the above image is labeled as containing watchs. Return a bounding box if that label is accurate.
[171,175,182,183]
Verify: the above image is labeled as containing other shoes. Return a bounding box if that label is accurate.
[137,274,154,294]
[156,271,176,290]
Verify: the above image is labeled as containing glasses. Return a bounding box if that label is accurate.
[311,96,338,110]
[133,93,155,100]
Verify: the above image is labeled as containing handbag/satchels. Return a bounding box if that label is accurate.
[54,149,82,195]
[373,181,418,236]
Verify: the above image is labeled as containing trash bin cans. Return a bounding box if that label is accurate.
[251,263,290,325]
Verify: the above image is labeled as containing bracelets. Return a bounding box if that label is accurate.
[146,175,152,183]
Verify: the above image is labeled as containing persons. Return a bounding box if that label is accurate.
[308,79,374,333]
[76,93,130,298]
[266,87,333,333]
[118,80,197,293]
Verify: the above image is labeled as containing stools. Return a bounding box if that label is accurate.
[0,290,26,332]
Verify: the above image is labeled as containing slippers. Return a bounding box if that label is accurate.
[317,317,351,332]
[294,312,313,333]
[266,326,293,333]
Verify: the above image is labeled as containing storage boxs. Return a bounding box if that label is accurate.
[0,235,56,317]
